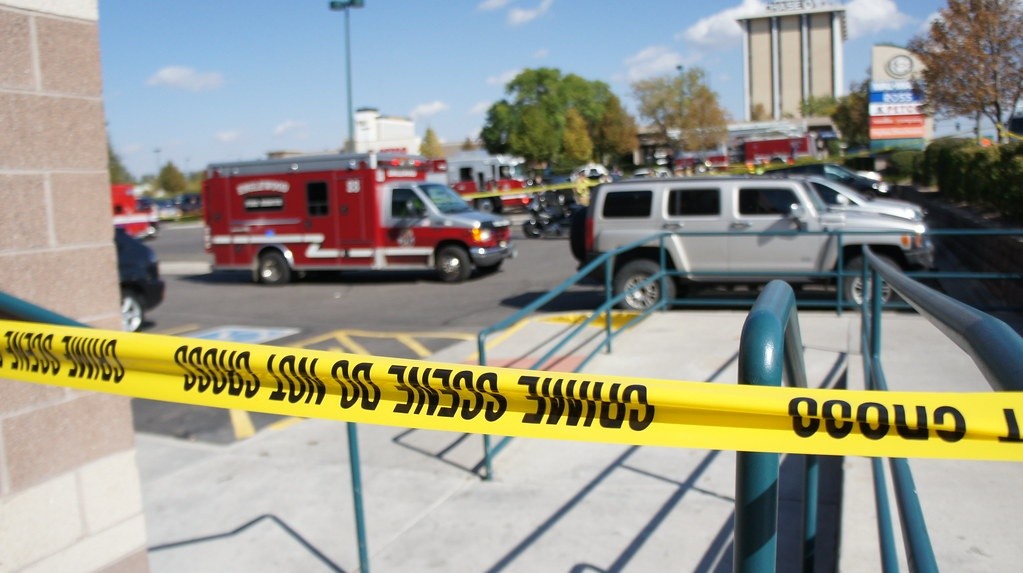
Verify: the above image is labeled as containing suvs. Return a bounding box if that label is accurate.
[568,166,935,312]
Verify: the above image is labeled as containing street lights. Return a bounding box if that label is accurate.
[330,0,363,153]
[676,65,684,119]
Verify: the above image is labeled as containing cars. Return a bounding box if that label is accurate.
[114,228,165,332]
[138,194,201,220]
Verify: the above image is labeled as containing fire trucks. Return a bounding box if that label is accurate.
[446,150,543,214]
[674,135,848,175]
[111,185,156,241]
[202,148,517,286]
